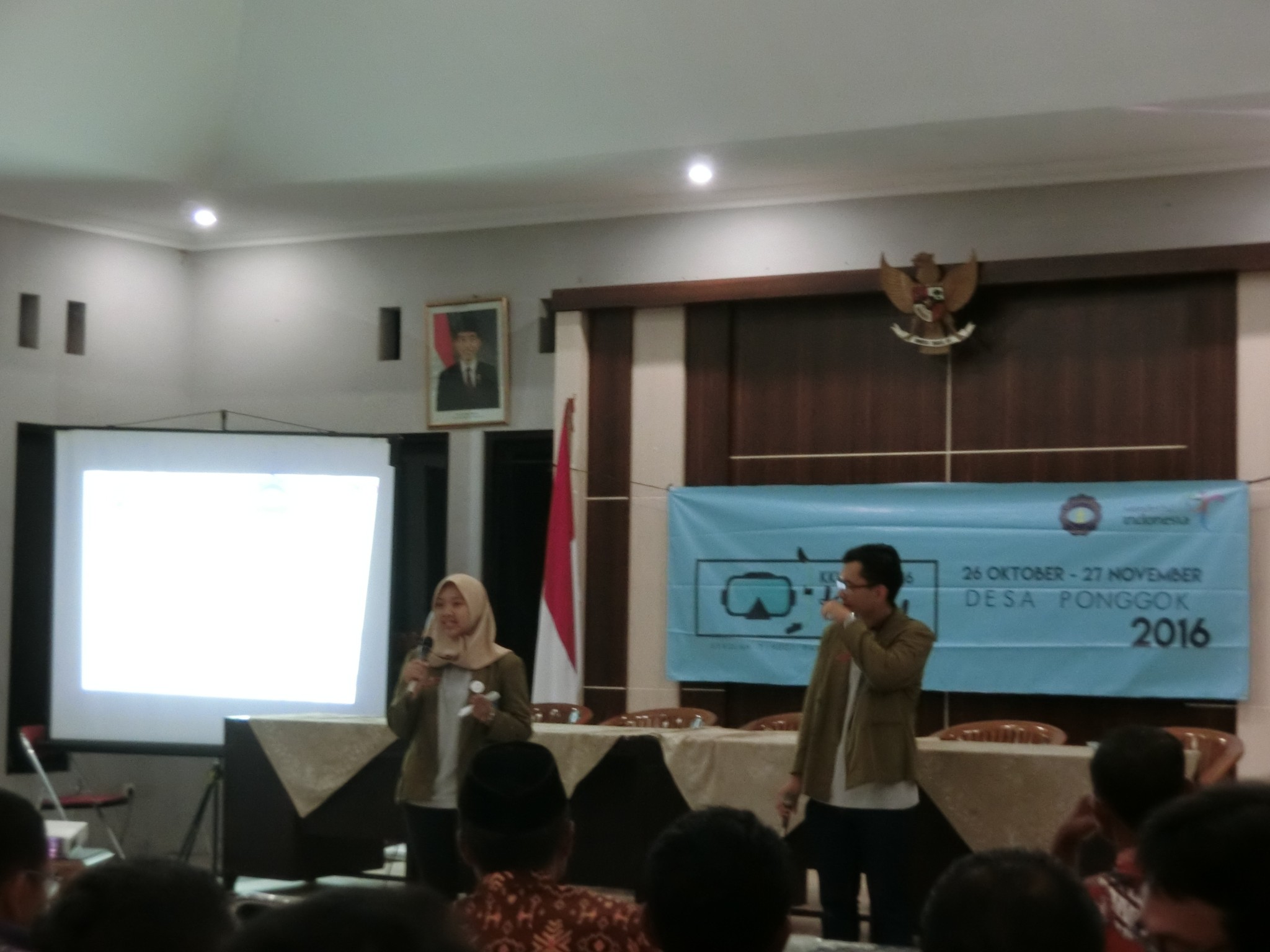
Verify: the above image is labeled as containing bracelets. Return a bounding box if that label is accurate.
[842,612,856,629]
[484,707,495,727]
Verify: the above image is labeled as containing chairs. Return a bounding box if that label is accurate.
[925,718,1074,750]
[739,708,810,731]
[1158,721,1251,792]
[526,697,601,731]
[17,722,137,859]
[595,709,744,732]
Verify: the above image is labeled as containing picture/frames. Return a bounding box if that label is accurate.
[425,293,509,430]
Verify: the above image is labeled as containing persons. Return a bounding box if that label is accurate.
[234,889,444,949]
[444,742,642,951]
[1053,727,1192,952]
[20,857,235,949]
[916,848,1106,952]
[392,575,530,894]
[1137,783,1270,952]
[775,542,935,946]
[1,788,85,951]
[435,313,498,412]
[641,804,793,951]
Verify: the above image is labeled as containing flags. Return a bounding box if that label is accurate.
[532,397,581,723]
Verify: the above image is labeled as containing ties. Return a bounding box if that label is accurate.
[466,367,473,387]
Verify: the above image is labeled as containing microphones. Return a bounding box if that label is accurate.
[780,787,796,832]
[407,635,433,699]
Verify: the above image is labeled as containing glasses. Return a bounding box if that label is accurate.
[836,579,874,591]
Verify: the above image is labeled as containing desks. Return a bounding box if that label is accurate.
[221,710,1197,919]
